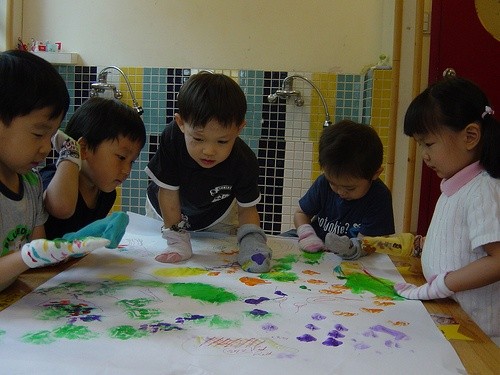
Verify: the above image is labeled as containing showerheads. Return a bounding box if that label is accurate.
[92,66,146,115]
[269,72,334,127]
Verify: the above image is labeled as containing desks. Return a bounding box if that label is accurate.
[0,254,500,375]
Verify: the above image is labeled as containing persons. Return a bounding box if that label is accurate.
[0,49,130,291]
[37,98,146,241]
[392,78,500,338]
[143,70,273,273]
[278,119,396,260]
